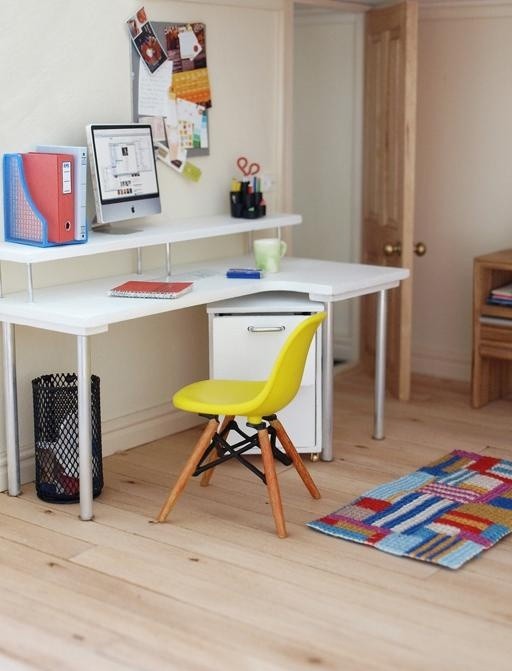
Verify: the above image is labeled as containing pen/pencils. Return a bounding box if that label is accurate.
[231,176,260,206]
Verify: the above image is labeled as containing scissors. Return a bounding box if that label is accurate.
[236,157,260,183]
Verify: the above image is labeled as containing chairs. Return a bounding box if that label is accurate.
[155,311,331,539]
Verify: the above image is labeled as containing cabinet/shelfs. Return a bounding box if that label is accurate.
[469,248,512,410]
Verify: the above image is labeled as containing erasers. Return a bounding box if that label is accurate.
[225,268,265,279]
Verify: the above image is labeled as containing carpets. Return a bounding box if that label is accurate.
[303,448,512,570]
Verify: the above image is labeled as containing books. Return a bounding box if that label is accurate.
[36,146,88,244]
[486,282,512,307]
[13,144,75,246]
[226,266,264,279]
[110,280,193,301]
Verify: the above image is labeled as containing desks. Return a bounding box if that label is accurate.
[0,212,412,522]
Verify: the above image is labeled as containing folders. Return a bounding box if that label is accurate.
[11,144,87,243]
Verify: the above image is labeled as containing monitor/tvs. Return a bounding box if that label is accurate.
[86,123,162,237]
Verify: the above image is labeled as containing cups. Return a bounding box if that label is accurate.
[253,237,288,273]
